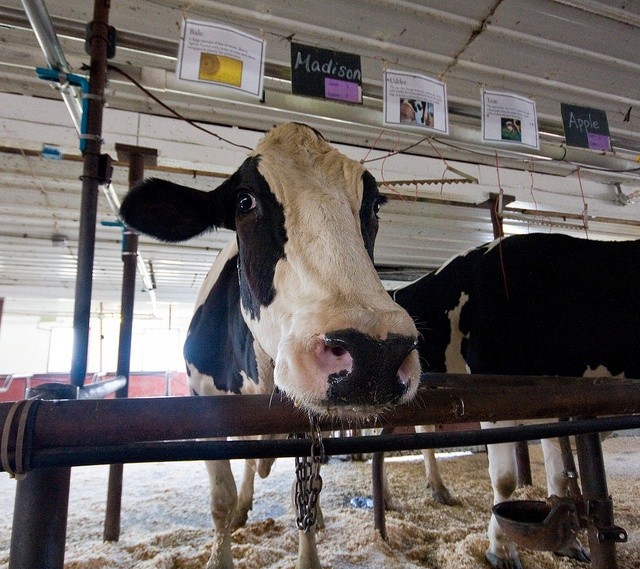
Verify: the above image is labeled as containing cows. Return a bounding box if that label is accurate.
[118,121,430,569]
[384,233,640,569]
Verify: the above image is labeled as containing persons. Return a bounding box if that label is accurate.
[503,121,519,141]
